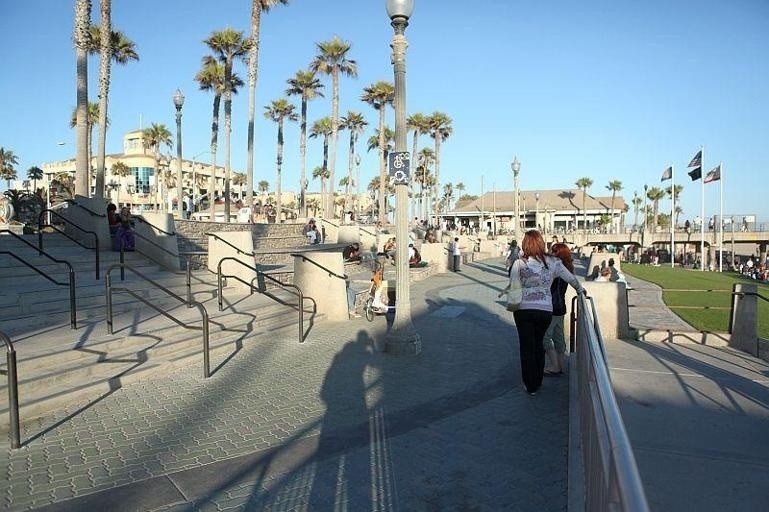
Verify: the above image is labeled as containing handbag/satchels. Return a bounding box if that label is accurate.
[505,277,523,311]
[551,287,563,310]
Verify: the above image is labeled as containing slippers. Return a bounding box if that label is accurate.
[544,368,563,376]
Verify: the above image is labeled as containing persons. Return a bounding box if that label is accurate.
[174,192,276,224]
[508,230,586,395]
[542,244,574,377]
[384,216,476,271]
[302,218,321,245]
[641,217,768,282]
[343,243,362,265]
[107,203,141,252]
[508,236,636,291]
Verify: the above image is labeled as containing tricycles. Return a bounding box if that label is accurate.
[363,260,396,322]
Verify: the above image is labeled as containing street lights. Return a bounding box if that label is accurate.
[511,157,521,243]
[191,152,211,205]
[173,89,185,218]
[523,192,526,226]
[534,191,540,212]
[385,0,422,357]
[57,141,77,145]
[543,202,550,254]
[644,183,648,225]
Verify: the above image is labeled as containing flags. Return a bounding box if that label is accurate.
[704,165,720,183]
[661,167,672,182]
[688,150,702,167]
[688,167,701,181]
